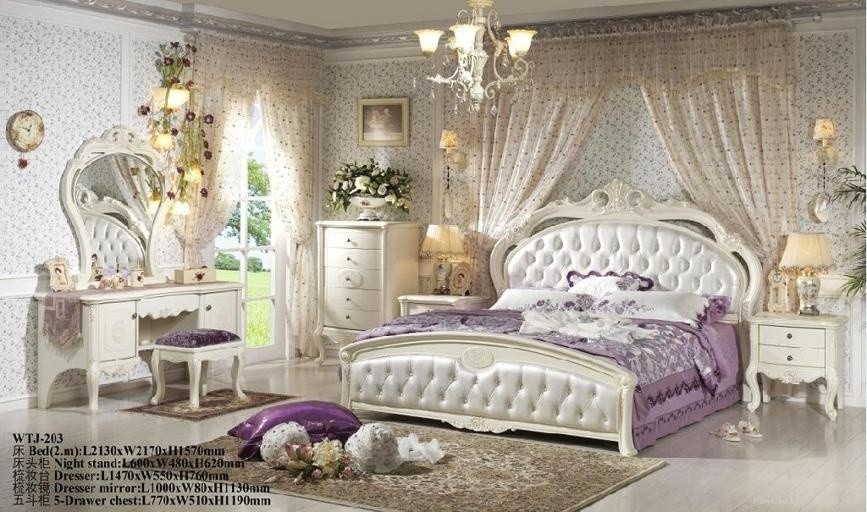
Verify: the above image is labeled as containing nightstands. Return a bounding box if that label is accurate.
[744,310,848,422]
[397,295,488,317]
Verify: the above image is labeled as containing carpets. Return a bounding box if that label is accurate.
[121,389,294,420]
[122,420,667,512]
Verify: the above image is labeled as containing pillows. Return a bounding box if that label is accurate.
[489,289,593,311]
[567,276,640,300]
[589,290,711,332]
[227,400,362,461]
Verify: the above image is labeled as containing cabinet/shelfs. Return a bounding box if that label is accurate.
[313,221,421,366]
[33,281,244,411]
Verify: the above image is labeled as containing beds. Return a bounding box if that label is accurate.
[339,177,765,459]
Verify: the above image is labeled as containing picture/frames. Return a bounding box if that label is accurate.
[357,98,409,146]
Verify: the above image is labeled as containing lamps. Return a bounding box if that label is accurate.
[813,118,837,165]
[413,0,538,117]
[439,128,465,163]
[419,224,464,294]
[778,232,834,315]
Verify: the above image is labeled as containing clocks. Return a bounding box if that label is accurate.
[6,110,44,168]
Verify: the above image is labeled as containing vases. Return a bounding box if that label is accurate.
[351,196,387,221]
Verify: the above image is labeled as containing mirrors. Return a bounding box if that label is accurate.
[59,125,174,291]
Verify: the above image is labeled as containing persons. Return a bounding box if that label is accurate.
[55,267,67,285]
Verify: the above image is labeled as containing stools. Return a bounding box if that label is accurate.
[150,329,249,410]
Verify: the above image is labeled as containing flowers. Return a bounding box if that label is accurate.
[136,41,214,200]
[323,158,413,215]
